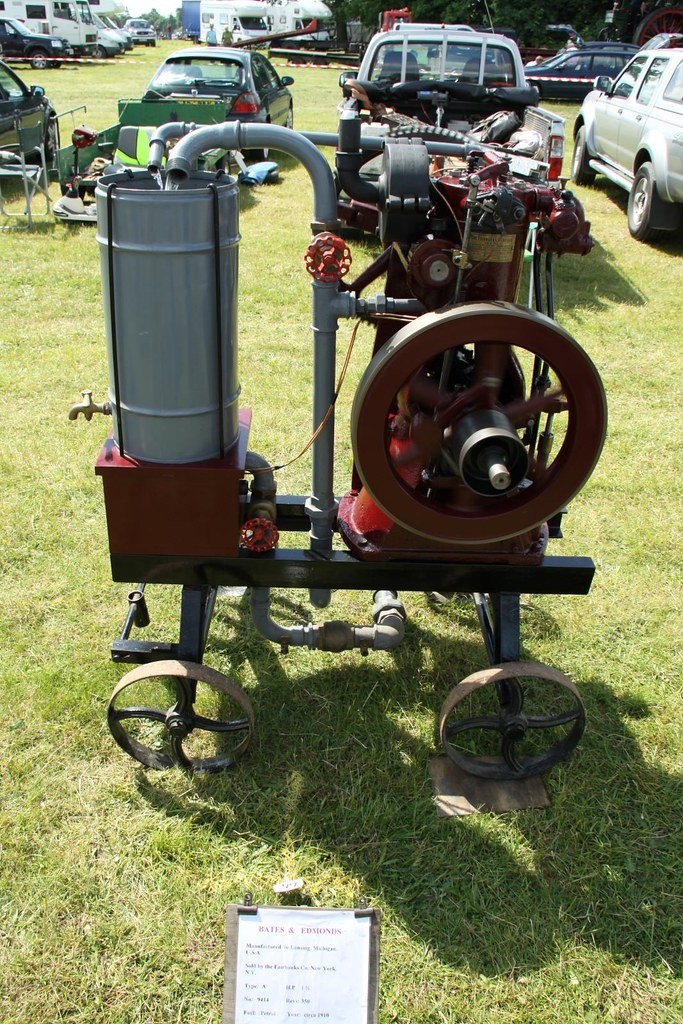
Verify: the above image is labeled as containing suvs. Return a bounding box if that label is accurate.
[569,47,683,246]
[0,18,75,69]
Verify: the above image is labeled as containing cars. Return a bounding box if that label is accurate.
[0,59,61,171]
[139,45,295,163]
[123,18,157,47]
[519,39,644,102]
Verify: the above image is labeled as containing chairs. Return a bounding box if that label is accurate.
[186,66,203,79]
[456,57,501,87]
[0,120,53,230]
[377,52,420,82]
[102,125,158,177]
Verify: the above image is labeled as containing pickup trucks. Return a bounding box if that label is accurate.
[317,23,568,216]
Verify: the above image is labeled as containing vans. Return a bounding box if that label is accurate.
[0,0,136,61]
